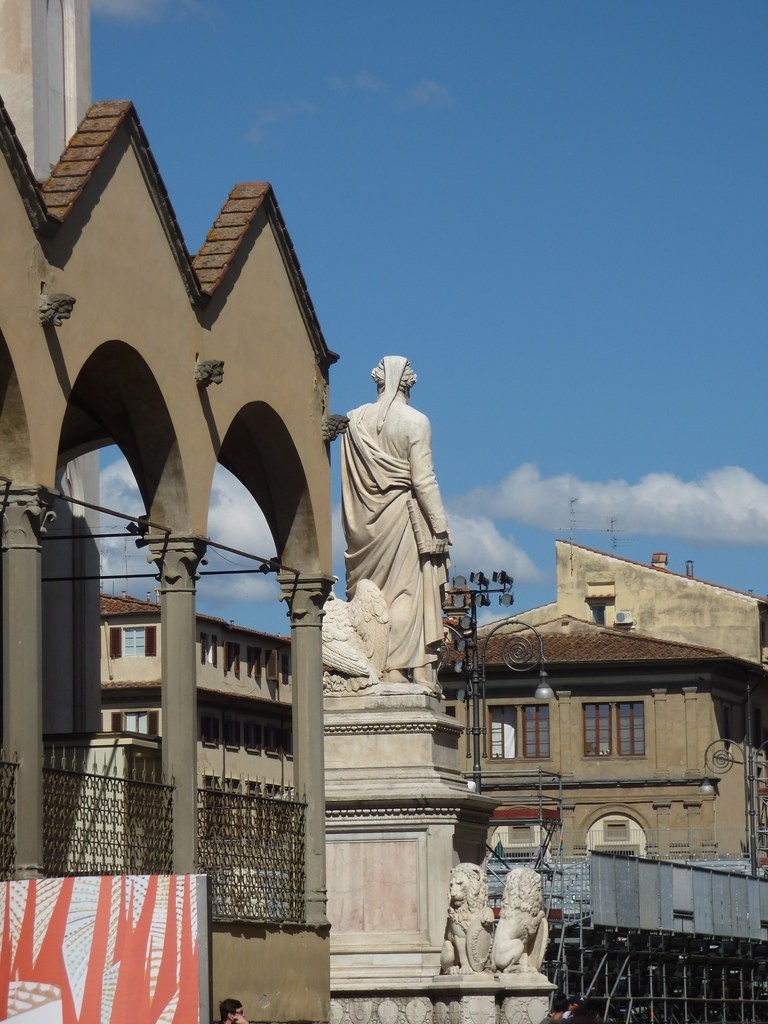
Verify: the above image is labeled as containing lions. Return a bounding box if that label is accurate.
[491,867,548,973]
[440,861,497,977]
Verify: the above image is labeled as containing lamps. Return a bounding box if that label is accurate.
[125,514,148,536]
[259,557,279,574]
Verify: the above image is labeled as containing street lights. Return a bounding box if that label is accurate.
[446,571,554,793]
[698,679,756,880]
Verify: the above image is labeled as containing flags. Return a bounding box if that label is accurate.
[495,842,508,863]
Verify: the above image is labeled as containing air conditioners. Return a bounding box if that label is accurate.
[615,611,633,625]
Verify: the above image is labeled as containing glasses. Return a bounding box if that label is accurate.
[234,1010,243,1015]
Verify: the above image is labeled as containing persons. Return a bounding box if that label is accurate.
[218,997,247,1024]
[341,356,454,697]
[540,992,601,1024]
